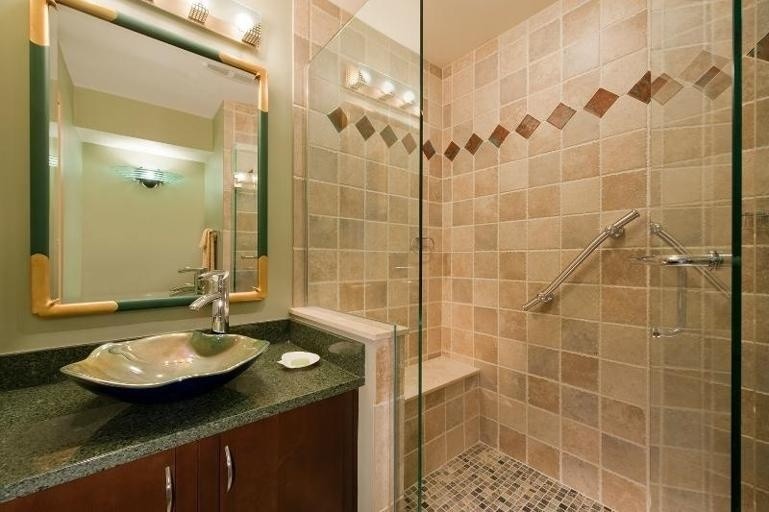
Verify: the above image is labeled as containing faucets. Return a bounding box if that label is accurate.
[187,268,230,334]
[167,265,206,298]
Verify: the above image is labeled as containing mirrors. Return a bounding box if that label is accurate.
[29,0,268,320]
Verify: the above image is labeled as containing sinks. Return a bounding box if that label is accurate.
[56,331,272,407]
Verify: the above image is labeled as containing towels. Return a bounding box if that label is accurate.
[199,228,216,271]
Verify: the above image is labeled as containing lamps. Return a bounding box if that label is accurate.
[347,69,416,110]
[188,0,262,48]
[129,149,167,190]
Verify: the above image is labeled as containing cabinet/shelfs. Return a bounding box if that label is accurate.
[0,387,359,512]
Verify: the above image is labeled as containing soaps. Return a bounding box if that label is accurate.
[289,354,310,366]
